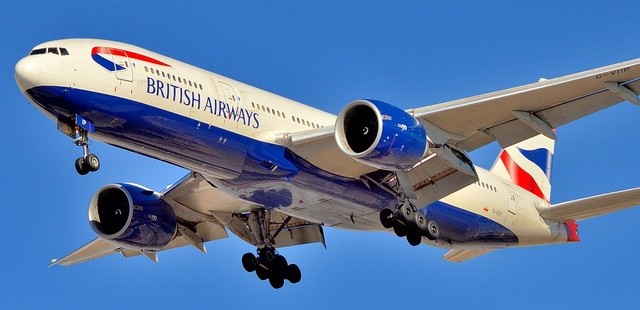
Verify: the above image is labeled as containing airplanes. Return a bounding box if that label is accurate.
[14,38,640,289]
